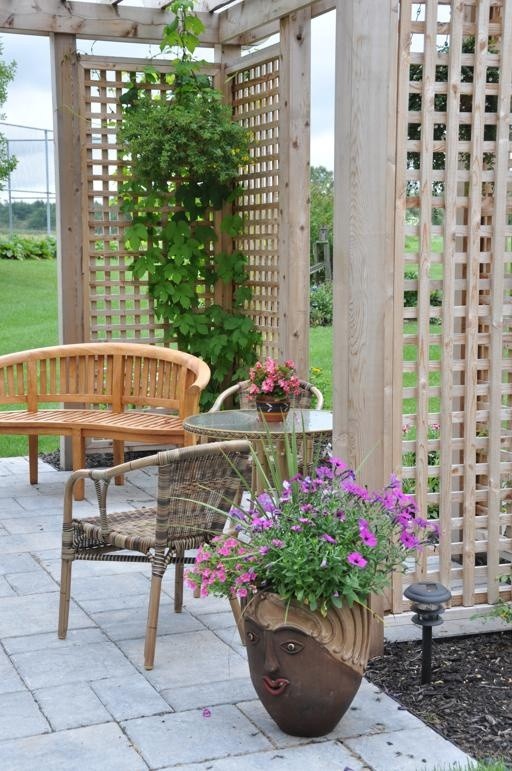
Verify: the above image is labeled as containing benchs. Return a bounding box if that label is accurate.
[0,343,211,501]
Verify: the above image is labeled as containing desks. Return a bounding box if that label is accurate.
[182,409,332,500]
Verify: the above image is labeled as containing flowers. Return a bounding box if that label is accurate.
[182,406,442,617]
[242,355,300,401]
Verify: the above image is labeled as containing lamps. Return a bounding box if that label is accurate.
[405,582,451,685]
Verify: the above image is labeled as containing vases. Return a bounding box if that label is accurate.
[239,590,370,738]
